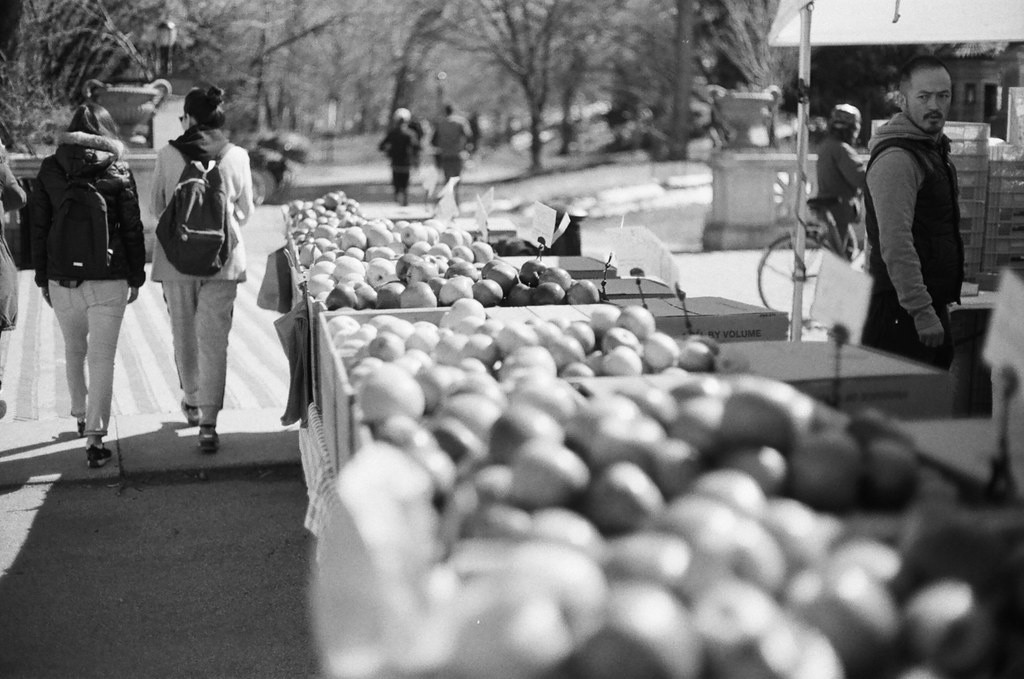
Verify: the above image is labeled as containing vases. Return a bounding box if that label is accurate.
[82,77,173,148]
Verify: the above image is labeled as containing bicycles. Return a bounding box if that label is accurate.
[756,193,874,328]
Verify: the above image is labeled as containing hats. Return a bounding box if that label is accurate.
[828,103,860,130]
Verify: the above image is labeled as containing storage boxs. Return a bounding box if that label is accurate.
[282,204,789,476]
[718,337,959,419]
[891,415,997,485]
[872,120,1023,289]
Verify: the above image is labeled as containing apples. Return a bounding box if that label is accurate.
[286,192,1023,679]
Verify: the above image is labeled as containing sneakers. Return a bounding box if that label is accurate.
[181,397,201,427]
[195,427,220,452]
[76,419,86,437]
[86,442,114,468]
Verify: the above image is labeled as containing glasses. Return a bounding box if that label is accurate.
[178,116,183,122]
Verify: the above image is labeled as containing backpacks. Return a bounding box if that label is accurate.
[43,160,123,280]
[152,142,240,278]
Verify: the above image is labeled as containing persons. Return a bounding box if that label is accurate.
[380,107,426,206]
[432,101,473,204]
[816,104,867,259]
[0,145,27,331]
[467,110,482,155]
[28,103,147,468]
[151,86,254,450]
[860,55,964,372]
